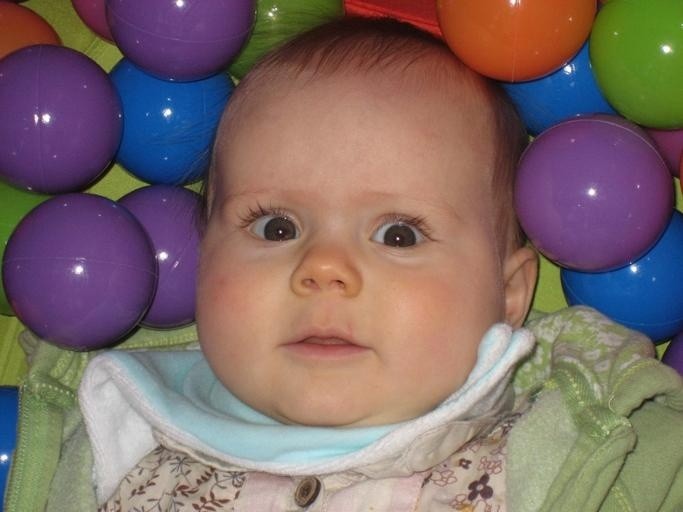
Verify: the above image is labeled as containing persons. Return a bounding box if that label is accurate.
[1,15,683,512]
